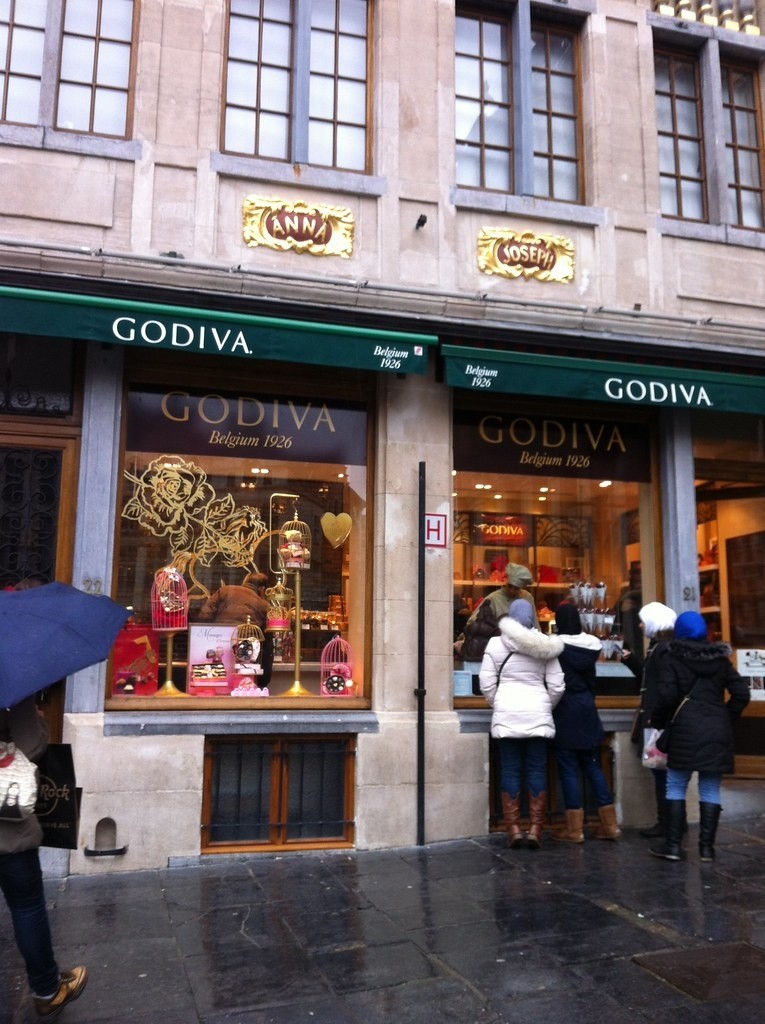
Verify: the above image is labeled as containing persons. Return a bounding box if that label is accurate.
[551,602,622,845]
[630,602,690,836]
[647,611,750,863]
[619,568,642,599]
[196,570,277,688]
[477,600,566,849]
[454,562,541,652]
[0,693,90,1015]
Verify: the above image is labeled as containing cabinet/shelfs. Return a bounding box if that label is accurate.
[698,565,721,615]
[453,579,577,622]
[283,618,343,663]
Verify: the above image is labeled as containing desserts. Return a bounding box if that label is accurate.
[116,676,136,694]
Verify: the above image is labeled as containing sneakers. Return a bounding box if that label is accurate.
[31,965,88,1021]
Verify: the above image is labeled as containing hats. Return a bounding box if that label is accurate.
[506,562,533,588]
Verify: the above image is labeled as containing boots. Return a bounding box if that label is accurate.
[527,791,547,849]
[549,808,585,842]
[698,801,722,861]
[592,804,621,838]
[639,788,667,836]
[502,793,524,847]
[648,799,685,860]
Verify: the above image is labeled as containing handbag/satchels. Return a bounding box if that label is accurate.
[0,741,39,821]
[656,721,673,754]
[642,727,668,770]
[34,744,83,849]
[630,709,645,744]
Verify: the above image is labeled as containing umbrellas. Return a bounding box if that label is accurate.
[1,579,134,709]
